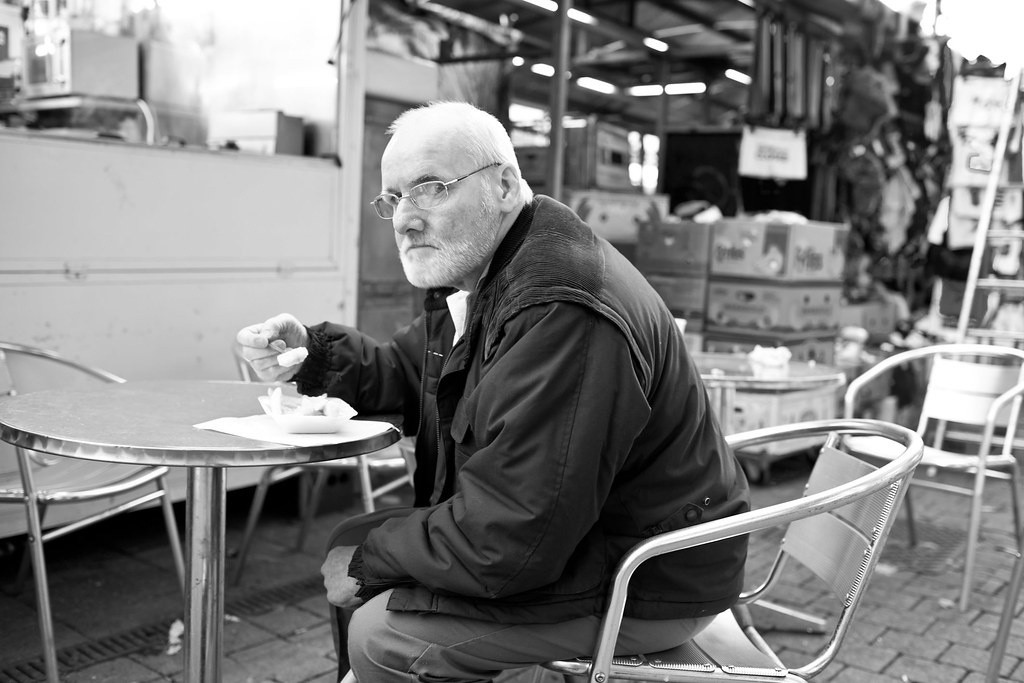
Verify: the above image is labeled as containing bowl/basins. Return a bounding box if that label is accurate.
[258,394,359,434]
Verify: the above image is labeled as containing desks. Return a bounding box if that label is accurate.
[0,378,402,683]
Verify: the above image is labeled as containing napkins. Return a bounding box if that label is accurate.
[193,416,401,447]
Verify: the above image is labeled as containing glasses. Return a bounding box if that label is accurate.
[370,162,501,219]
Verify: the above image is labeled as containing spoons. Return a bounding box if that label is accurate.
[267,343,309,367]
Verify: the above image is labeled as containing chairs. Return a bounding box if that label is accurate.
[337,419,924,683]
[843,345,1024,614]
[232,343,418,587]
[0,340,185,683]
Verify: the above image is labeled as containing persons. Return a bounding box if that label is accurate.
[237,103,750,683]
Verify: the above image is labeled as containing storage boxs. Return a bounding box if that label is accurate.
[697,372,841,455]
[706,279,839,342]
[708,217,851,285]
[638,220,713,277]
[513,146,548,186]
[561,187,670,245]
[641,273,706,318]
[703,327,837,367]
